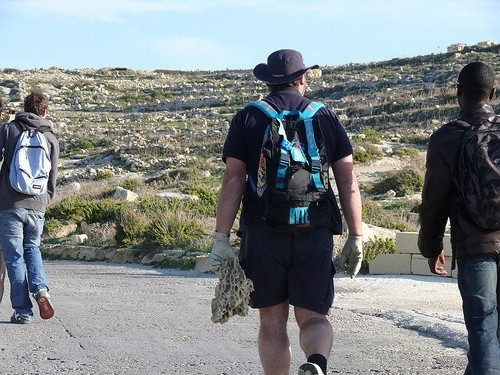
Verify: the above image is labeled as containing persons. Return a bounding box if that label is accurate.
[208,49,362,375]
[417,62,500,374]
[1,92,60,325]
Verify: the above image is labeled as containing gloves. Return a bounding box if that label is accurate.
[208,230,237,274]
[340,234,364,279]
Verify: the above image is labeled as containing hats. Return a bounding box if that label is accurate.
[253,49,320,84]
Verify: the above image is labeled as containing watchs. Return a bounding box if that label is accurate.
[213,229,230,238]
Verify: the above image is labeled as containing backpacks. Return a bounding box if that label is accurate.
[10,120,53,196]
[456,111,500,230]
[245,100,329,233]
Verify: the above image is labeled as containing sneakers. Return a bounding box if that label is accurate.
[10,310,32,324]
[298,362,324,375]
[35,289,54,319]
